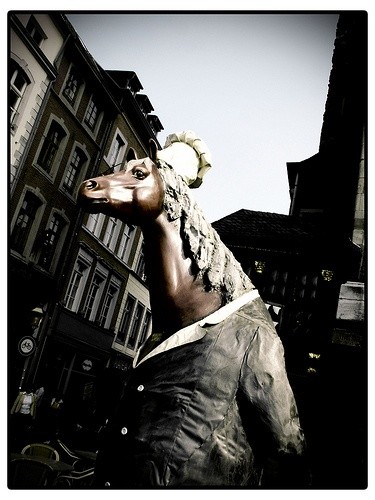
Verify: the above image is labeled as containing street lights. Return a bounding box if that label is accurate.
[17,298,52,395]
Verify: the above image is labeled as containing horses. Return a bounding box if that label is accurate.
[75,139,255,367]
